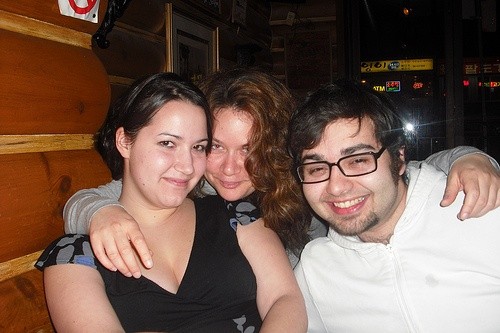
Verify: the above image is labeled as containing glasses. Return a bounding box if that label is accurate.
[297,143,387,184]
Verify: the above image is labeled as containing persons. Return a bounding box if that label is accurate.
[293,83,500,333]
[34,73,308,333]
[62,67,500,280]
